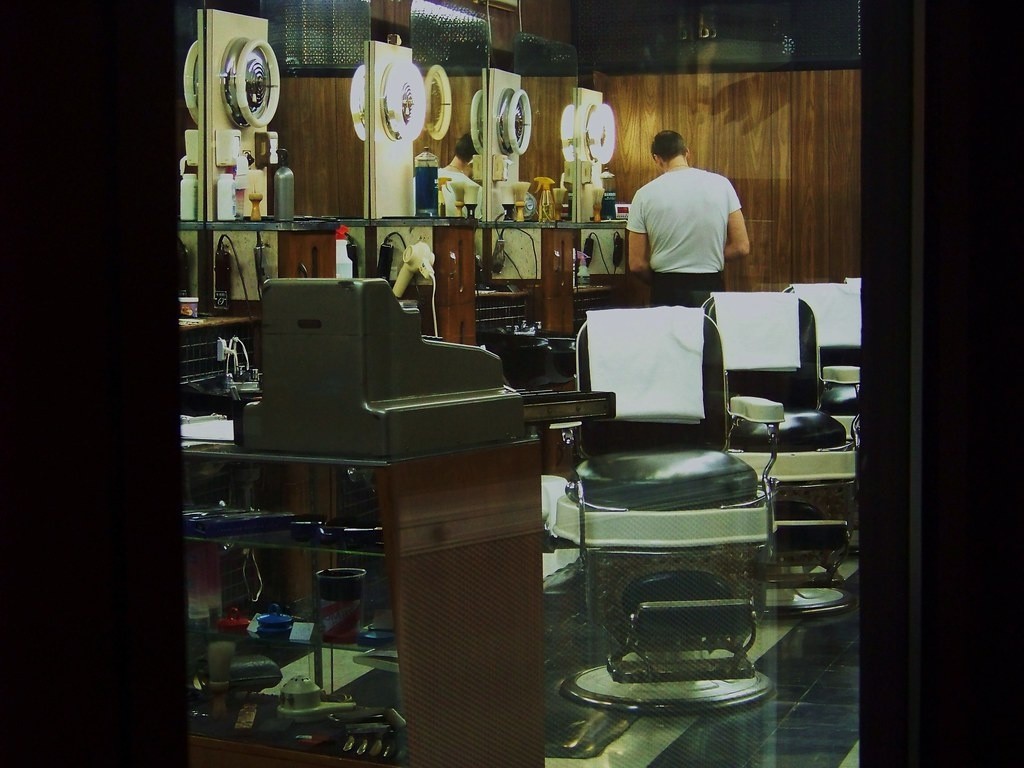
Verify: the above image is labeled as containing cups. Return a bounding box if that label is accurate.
[315,567,367,645]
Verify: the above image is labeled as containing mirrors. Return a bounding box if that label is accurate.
[410,0,491,222]
[207,0,371,218]
[515,32,577,220]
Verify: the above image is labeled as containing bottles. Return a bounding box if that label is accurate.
[217,173,236,221]
[273,148,295,222]
[413,146,439,218]
[180,173,198,221]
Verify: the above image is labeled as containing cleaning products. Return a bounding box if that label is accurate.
[273,149,294,220]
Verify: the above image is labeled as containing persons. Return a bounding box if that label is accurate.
[626,130,749,307]
[413,133,504,287]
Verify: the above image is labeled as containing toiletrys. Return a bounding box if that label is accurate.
[534,176,556,222]
[216,173,236,221]
[180,174,198,220]
[438,177,452,218]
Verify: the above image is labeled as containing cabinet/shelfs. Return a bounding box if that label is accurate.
[182,438,544,768]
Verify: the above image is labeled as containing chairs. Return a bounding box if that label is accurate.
[554,306,785,717]
[784,280,863,555]
[702,291,859,619]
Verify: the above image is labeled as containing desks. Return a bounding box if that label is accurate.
[202,219,628,230]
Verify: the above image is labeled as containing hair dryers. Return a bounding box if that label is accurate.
[392,241,435,298]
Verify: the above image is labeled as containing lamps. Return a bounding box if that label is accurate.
[471,90,483,154]
[497,88,531,155]
[349,64,365,141]
[426,64,456,141]
[585,101,616,165]
[560,103,574,162]
[220,36,280,129]
[379,60,426,142]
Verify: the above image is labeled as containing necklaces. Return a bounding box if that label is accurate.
[448,162,464,173]
[667,165,687,170]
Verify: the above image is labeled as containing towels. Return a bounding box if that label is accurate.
[710,291,801,372]
[585,305,706,426]
[789,282,862,348]
